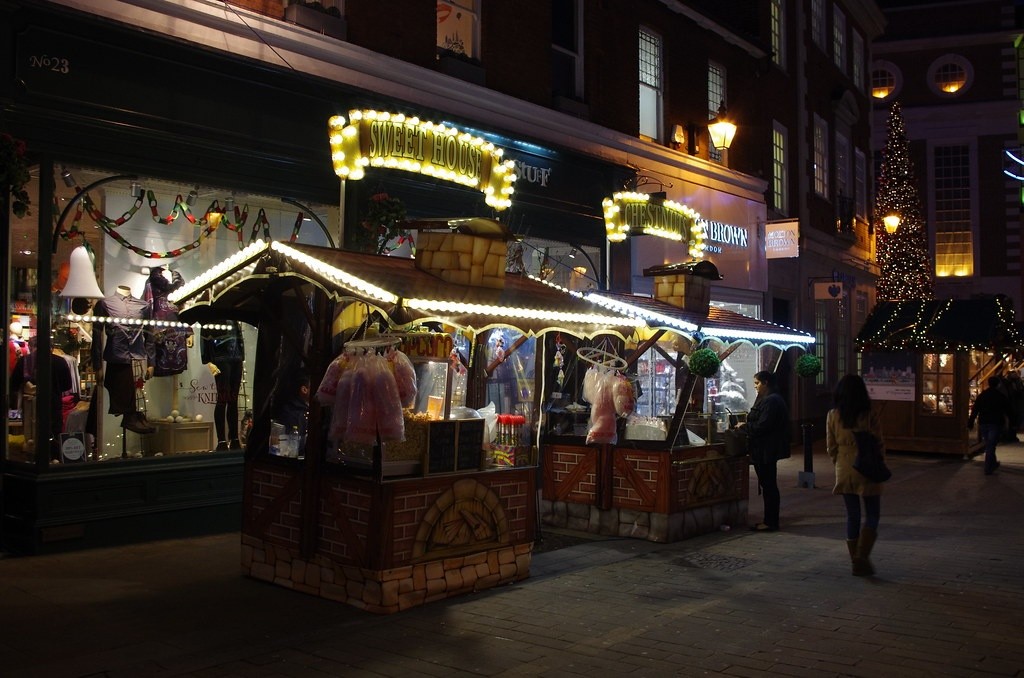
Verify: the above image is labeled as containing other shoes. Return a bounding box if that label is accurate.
[992,462,1000,468]
[137,412,156,433]
[216,442,228,452]
[229,440,241,450]
[120,412,150,434]
[749,523,779,532]
[984,464,992,475]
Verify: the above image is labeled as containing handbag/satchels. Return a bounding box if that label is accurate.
[724,427,747,456]
[852,407,892,484]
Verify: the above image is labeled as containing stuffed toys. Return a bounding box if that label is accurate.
[583,368,636,444]
[317,350,417,443]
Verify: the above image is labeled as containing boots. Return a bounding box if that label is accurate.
[846,525,879,578]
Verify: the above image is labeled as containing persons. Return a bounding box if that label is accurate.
[200,320,245,451]
[735,371,792,533]
[967,370,1024,474]
[826,374,885,576]
[91,286,156,413]
[10,335,72,464]
[278,374,310,435]
[60,342,82,397]
